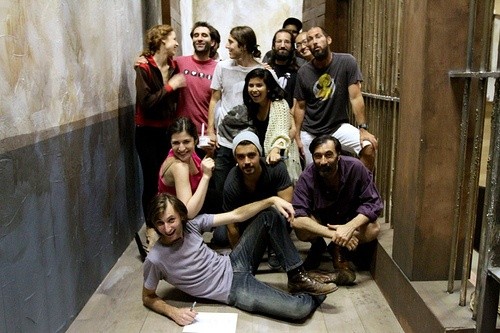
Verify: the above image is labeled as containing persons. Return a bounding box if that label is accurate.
[134,117,215,263]
[291,135,383,284]
[295,31,314,62]
[207,26,279,214]
[142,193,356,326]
[294,26,378,172]
[224,130,294,252]
[243,68,299,268]
[266,29,301,110]
[134,20,272,161]
[262,18,302,65]
[133,25,186,244]
[209,26,227,63]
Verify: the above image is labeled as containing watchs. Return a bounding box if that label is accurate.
[358,122,368,130]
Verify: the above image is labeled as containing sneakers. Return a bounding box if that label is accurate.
[267,246,282,270]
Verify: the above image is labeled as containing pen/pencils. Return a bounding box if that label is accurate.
[190,301,196,311]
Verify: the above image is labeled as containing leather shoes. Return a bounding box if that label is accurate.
[288,272,338,295]
[308,269,356,284]
[327,241,352,269]
[303,240,326,270]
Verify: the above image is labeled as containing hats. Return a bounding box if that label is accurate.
[233,127,262,156]
[283,18,303,30]
